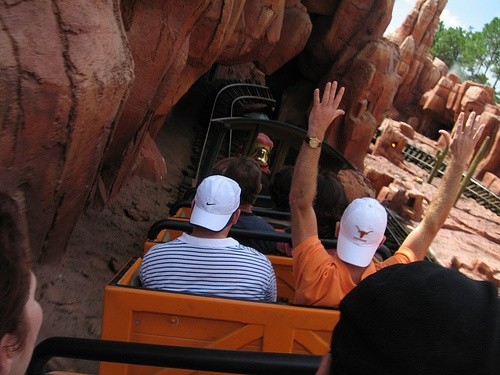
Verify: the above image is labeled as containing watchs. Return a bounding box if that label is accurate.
[304,135,322,149]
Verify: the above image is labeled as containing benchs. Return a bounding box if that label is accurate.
[99,195,391,375]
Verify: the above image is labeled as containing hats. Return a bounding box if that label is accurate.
[336,196,388,268]
[332,261,498,375]
[189,175,241,232]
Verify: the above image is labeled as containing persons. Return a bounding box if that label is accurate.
[289,81,486,309]
[211,156,277,255]
[277,171,350,257]
[137,175,277,303]
